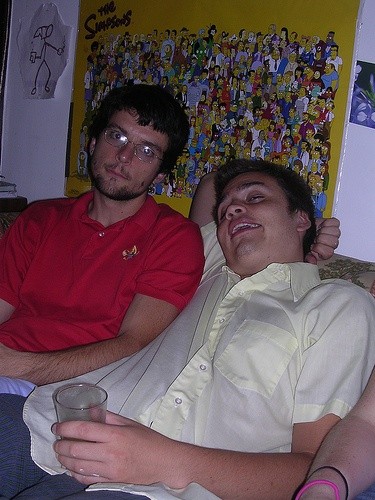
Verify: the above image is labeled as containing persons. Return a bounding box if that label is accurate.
[0,159,375,500]
[294,362,375,500]
[0,83,205,397]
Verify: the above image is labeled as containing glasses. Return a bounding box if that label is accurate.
[104,128,163,163]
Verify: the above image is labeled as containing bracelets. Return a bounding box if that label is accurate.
[303,465,349,500]
[295,480,340,500]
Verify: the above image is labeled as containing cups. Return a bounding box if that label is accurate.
[54,382,109,439]
